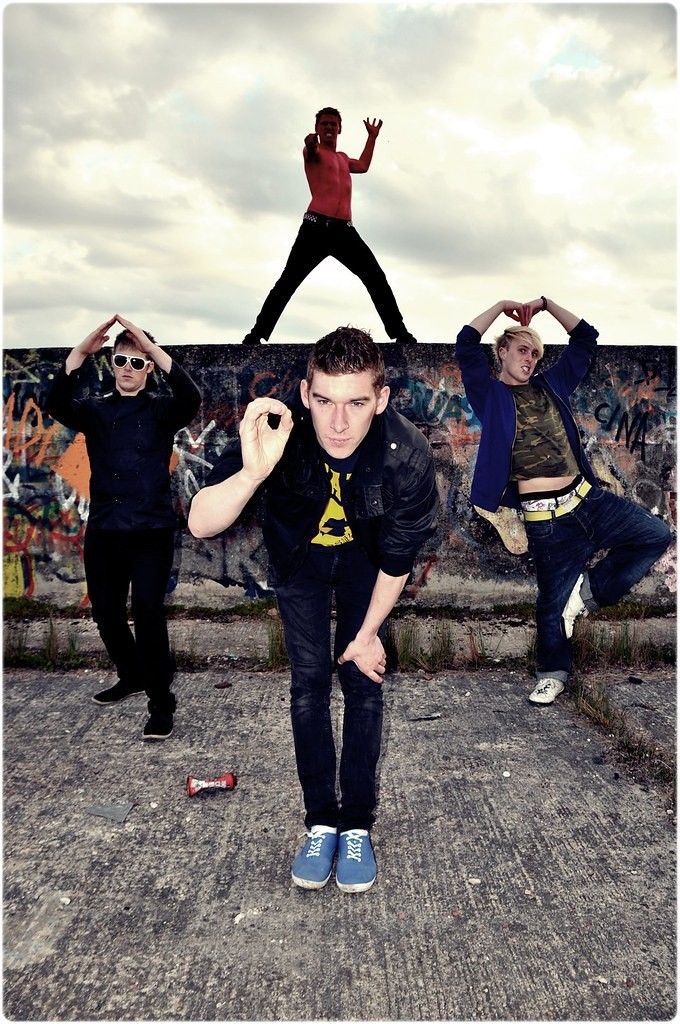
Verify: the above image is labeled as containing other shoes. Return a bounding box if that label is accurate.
[91,680,146,705]
[243,329,264,347]
[141,711,173,739]
[396,333,417,345]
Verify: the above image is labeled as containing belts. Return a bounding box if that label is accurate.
[525,481,592,522]
[304,212,353,228]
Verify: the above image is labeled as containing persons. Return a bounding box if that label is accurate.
[45,315,204,739]
[456,295,669,705]
[187,325,441,893]
[242,107,417,343]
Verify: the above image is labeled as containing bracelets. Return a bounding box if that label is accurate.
[540,295,547,311]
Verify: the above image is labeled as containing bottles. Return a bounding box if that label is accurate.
[186,772,237,796]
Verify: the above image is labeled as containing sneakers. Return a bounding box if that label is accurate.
[336,829,377,893]
[292,828,338,890]
[561,572,589,640]
[529,677,565,705]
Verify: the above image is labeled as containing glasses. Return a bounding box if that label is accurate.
[112,354,151,371]
